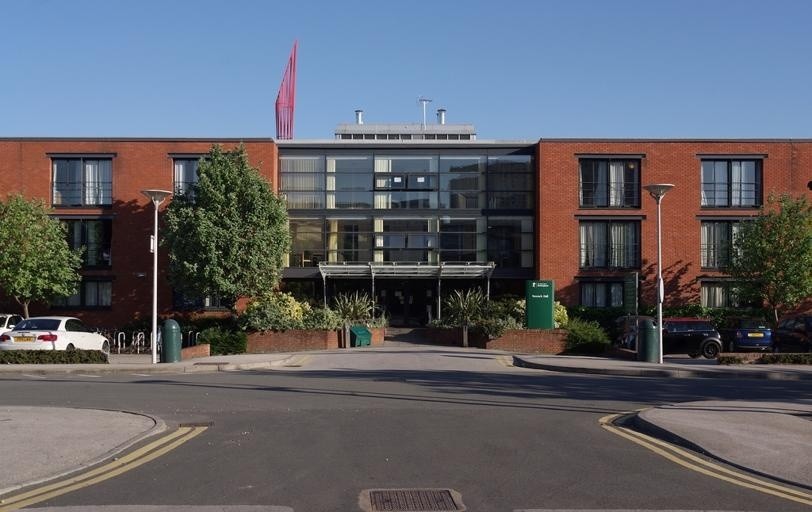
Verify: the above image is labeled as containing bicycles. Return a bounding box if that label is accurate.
[126,328,149,355]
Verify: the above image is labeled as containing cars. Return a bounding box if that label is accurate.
[612,314,671,357]
[0,315,110,356]
[0,312,25,337]
[777,315,812,352]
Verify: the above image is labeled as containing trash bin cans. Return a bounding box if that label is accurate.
[160,319,181,363]
[639,319,660,363]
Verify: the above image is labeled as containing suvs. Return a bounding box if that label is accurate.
[718,310,777,353]
[657,316,722,359]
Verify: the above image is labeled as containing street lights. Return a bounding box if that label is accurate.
[641,182,675,363]
[140,187,172,363]
